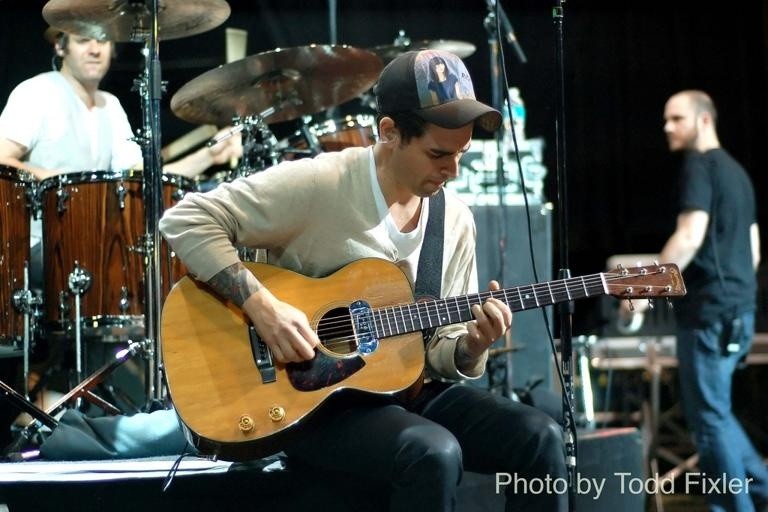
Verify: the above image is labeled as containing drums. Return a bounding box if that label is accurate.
[37,170,197,338]
[1,163,37,358]
[274,115,378,166]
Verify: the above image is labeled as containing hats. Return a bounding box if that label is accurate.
[376,50,503,134]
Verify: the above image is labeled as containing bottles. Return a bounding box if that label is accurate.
[503,88,526,141]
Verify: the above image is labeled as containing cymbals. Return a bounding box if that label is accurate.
[42,0,230,42]
[169,38,476,125]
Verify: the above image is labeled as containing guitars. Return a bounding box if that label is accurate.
[159,258,686,460]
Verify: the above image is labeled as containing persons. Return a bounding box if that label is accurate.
[158,46,569,511]
[0,22,144,291]
[426,54,461,105]
[620,90,768,512]
